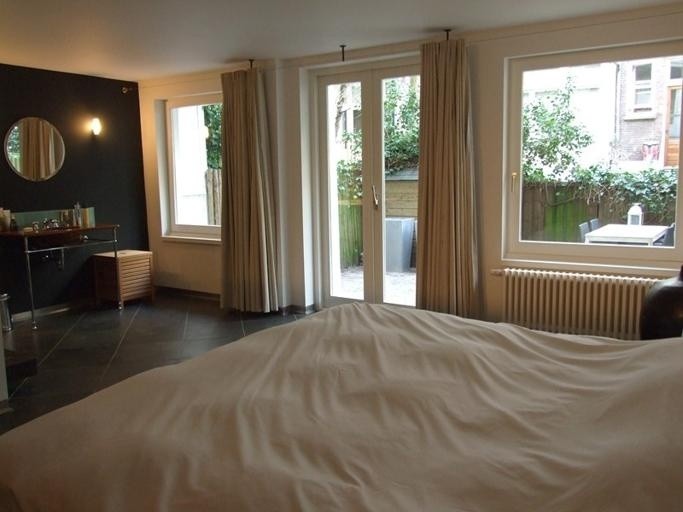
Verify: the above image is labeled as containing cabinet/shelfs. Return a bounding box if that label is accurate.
[93,248,155,309]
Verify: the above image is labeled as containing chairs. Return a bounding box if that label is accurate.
[579,222,591,241]
[657,222,675,242]
[654,226,674,246]
[590,218,601,230]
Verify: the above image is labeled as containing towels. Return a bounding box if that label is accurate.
[20,117,56,180]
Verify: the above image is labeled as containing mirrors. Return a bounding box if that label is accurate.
[4,116,67,182]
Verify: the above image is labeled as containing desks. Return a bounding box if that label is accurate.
[1,224,124,330]
[584,223,670,246]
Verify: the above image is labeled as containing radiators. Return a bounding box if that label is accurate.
[491,266,673,340]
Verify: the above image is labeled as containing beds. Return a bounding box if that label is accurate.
[0,297,683,512]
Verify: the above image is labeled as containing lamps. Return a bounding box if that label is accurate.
[91,115,103,136]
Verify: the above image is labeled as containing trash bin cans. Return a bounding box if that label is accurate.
[0,293,14,332]
[385,217,415,273]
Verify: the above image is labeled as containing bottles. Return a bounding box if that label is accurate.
[628,202,642,231]
[73,202,82,226]
[10,216,16,233]
